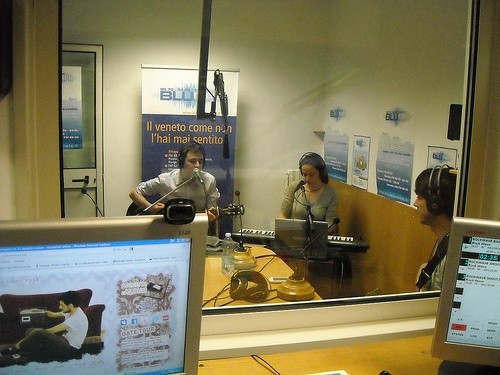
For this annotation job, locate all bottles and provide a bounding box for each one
[221,233,234,275]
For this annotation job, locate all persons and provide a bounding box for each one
[412,167,458,295]
[277,150,339,232]
[0,292,88,366]
[128,144,220,221]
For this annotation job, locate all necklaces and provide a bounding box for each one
[305,182,324,192]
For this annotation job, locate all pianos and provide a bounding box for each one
[231,228,371,297]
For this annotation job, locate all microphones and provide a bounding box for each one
[193,167,204,182]
[289,216,339,279]
[81,188,105,216]
[234,189,246,251]
[297,178,307,188]
[224,126,230,159]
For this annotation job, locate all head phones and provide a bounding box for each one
[427,161,450,214]
[299,150,326,170]
[177,142,200,159]
[229,268,272,300]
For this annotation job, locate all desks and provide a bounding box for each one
[202,244,323,307]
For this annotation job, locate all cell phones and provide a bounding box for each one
[269,276,288,283]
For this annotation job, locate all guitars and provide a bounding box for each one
[125,198,245,218]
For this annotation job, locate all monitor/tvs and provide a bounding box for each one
[429,216,500,374]
[0,210,208,375]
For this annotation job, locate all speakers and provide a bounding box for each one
[447,103,462,140]
